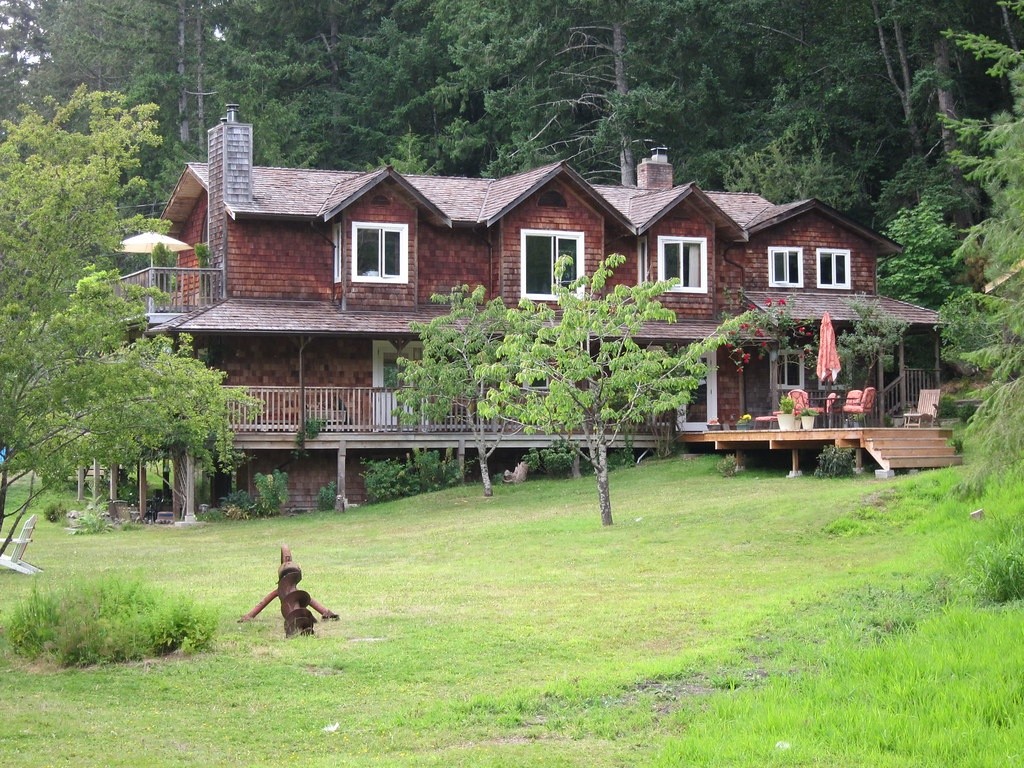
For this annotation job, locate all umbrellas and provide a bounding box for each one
[114,231,193,268]
[817,311,841,395]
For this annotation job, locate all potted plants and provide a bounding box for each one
[776,394,795,430]
[795,407,820,430]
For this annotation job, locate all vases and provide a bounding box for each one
[735,423,751,430]
[707,424,722,431]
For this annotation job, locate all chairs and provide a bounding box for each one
[789,389,825,428]
[753,411,783,429]
[808,393,836,428]
[834,390,863,428]
[843,387,876,428]
[0,514,45,576]
[902,389,942,429]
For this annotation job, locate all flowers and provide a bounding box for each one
[707,417,722,424]
[737,414,751,423]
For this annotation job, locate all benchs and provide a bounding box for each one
[307,410,347,433]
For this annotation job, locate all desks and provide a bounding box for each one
[807,397,859,428]
